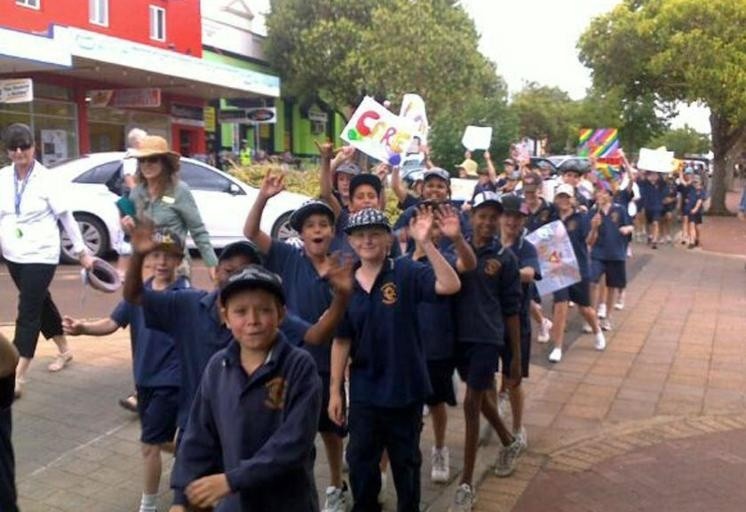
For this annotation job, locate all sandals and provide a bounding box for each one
[120,392,138,411]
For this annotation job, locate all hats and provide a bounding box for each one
[219,263,286,305]
[87,260,122,292]
[343,208,390,234]
[334,158,612,207]
[220,241,264,265]
[289,199,335,229]
[143,231,182,255]
[123,136,180,171]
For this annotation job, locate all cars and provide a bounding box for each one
[43,147,324,263]
[683,153,714,200]
[448,156,638,201]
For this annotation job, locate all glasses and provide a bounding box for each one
[7,143,32,151]
[138,157,160,163]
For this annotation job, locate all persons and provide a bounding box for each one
[121,127,149,191]
[0,122,95,400]
[122,200,354,512]
[114,136,222,416]
[61,231,194,511]
[169,264,323,511]
[244,133,642,510]
[634,158,708,249]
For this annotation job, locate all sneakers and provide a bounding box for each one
[537,318,552,343]
[430,446,449,482]
[494,435,527,476]
[48,352,72,371]
[15,379,26,397]
[323,481,353,512]
[583,299,623,349]
[447,483,477,512]
[549,348,562,361]
